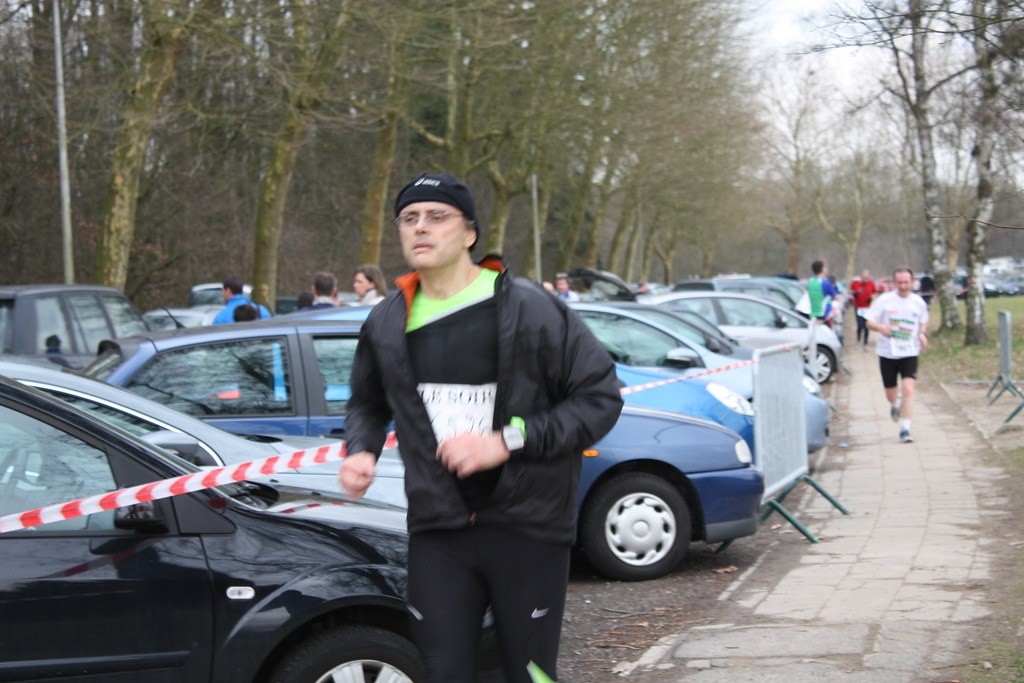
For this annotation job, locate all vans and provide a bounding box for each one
[0,284,155,376]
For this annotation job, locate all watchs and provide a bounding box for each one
[502,425,524,451]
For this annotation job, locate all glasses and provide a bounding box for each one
[394,210,464,225]
[555,272,568,277]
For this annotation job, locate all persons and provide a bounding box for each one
[634,280,650,295]
[866,267,929,443]
[212,263,389,326]
[807,260,935,352]
[550,272,580,302]
[339,173,624,683]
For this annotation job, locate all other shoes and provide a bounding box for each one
[898,427,914,443]
[890,403,899,423]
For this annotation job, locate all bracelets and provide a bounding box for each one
[920,329,926,334]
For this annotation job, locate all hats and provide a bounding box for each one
[393,171,478,221]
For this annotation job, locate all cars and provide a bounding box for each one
[0,377,441,683]
[0,357,413,512]
[135,267,1022,458]
[74,316,766,583]
